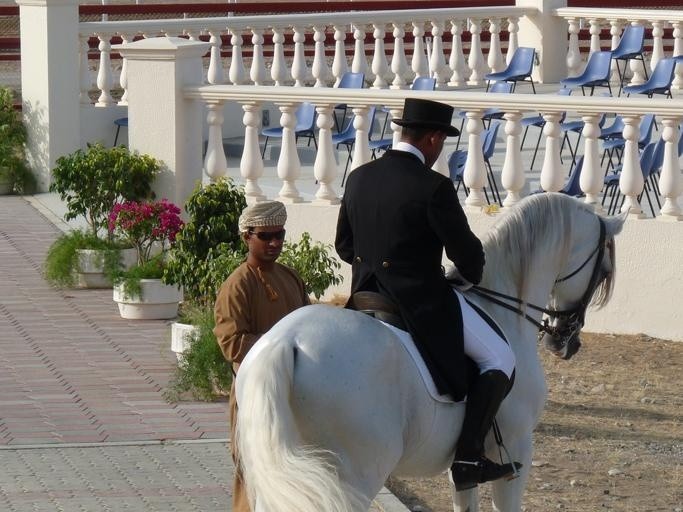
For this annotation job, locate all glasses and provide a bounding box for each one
[249,229,285,241]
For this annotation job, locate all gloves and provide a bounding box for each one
[445,267,474,292]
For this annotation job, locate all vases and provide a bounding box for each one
[110,273,181,320]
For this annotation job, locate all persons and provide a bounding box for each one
[212,201,312,512]
[334,125,523,492]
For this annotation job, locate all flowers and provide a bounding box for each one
[105,200,187,301]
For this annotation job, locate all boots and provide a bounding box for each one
[450,455,523,492]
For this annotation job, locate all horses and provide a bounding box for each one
[228,187,633,512]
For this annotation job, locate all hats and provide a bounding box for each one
[391,99,460,137]
[237,200,286,232]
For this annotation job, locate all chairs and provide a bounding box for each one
[113,117,128,145]
[261,24,683,219]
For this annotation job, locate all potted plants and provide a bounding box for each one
[43,142,157,291]
[1,84,37,197]
[163,175,344,400]
